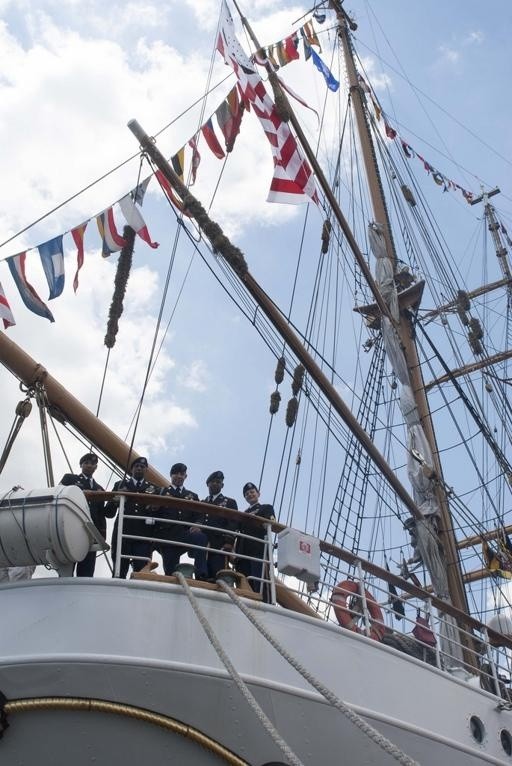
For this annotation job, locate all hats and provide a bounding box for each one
[170,463,187,475]
[80,454,98,464]
[131,457,147,469]
[243,482,257,496]
[205,471,224,484]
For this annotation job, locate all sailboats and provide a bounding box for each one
[1,1,512,766]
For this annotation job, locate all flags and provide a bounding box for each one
[309,46,340,93]
[215,0,320,207]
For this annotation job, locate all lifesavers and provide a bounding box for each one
[332,581,384,644]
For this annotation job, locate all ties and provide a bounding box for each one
[176,487,181,494]
[136,482,141,488]
[208,496,213,504]
[87,478,91,489]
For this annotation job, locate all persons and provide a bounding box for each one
[151,463,217,583]
[200,471,238,584]
[58,453,106,577]
[104,457,159,579]
[235,483,275,604]
